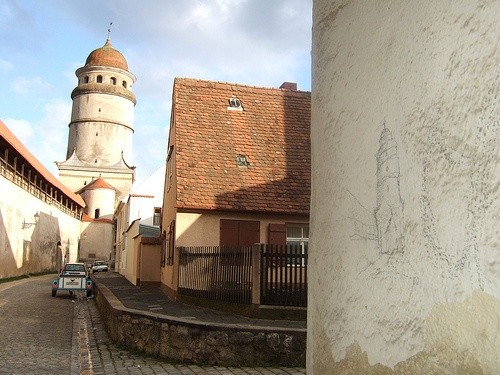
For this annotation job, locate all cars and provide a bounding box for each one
[92,260,108,273]
[51,262,93,297]
